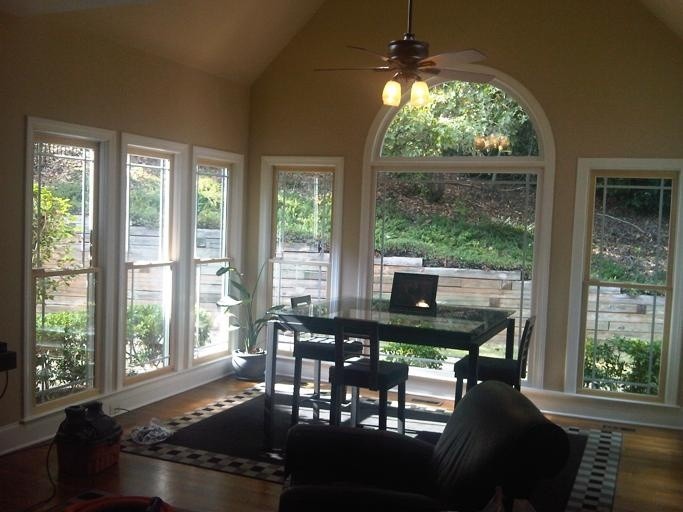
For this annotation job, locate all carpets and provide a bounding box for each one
[120,381,624,512]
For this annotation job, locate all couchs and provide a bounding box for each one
[276,378,569,512]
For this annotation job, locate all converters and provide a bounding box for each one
[0,351,16,371]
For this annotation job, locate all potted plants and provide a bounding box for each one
[214,256,288,382]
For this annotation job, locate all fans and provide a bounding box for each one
[311,0,495,85]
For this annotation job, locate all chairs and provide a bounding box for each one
[451,316,536,410]
[290,294,362,427]
[326,316,408,435]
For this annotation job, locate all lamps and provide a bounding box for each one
[380,69,431,107]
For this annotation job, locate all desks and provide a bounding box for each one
[263,296,516,457]
